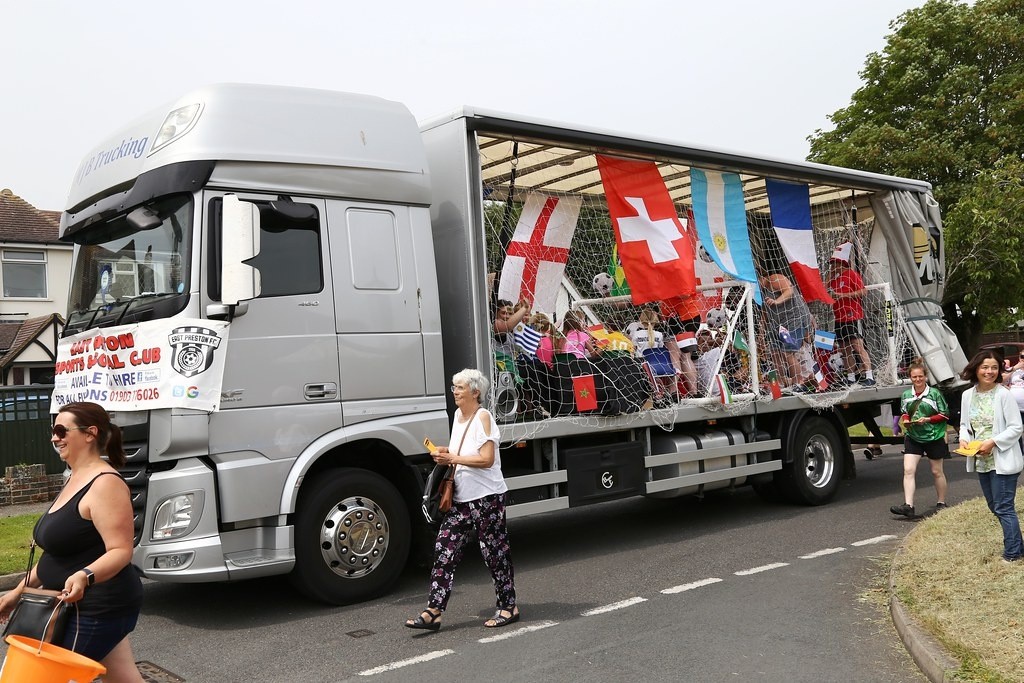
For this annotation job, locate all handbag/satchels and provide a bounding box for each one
[438,477,452,512]
[421,464,449,523]
[2,593,67,645]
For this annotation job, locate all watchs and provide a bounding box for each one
[81,569,94,586]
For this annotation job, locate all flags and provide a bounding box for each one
[594,154,763,305]
[571,375,597,412]
[769,370,782,399]
[733,331,751,357]
[765,177,836,304]
[497,194,582,315]
[716,374,733,405]
[815,330,836,351]
[514,322,541,354]
[587,323,609,347]
[778,327,795,345]
[812,364,828,389]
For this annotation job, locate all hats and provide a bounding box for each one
[826,240,853,266]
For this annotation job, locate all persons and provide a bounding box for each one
[0,402,147,683]
[494,242,873,411]
[864,430,883,460]
[892,403,904,436]
[890,358,948,517]
[404,368,518,629]
[953,351,1024,561]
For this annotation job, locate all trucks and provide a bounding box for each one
[50,84,941,607]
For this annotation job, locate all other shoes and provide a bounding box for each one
[864,447,874,460]
[792,379,817,394]
[814,380,843,392]
[523,410,548,421]
[652,397,671,409]
[667,391,682,405]
[688,392,704,399]
[890,503,915,517]
[857,379,878,388]
[873,447,882,455]
[932,502,947,516]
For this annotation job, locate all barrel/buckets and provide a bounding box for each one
[0,592,107,683]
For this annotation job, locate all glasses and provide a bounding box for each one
[51,424,104,439]
[450,385,477,394]
[1020,355,1024,359]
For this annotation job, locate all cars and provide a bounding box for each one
[979,342,1024,368]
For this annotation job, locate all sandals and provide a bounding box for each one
[484,603,520,627]
[404,610,441,631]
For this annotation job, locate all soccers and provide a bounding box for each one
[625,322,645,336]
[706,307,729,330]
[592,272,616,294]
[827,353,845,371]
